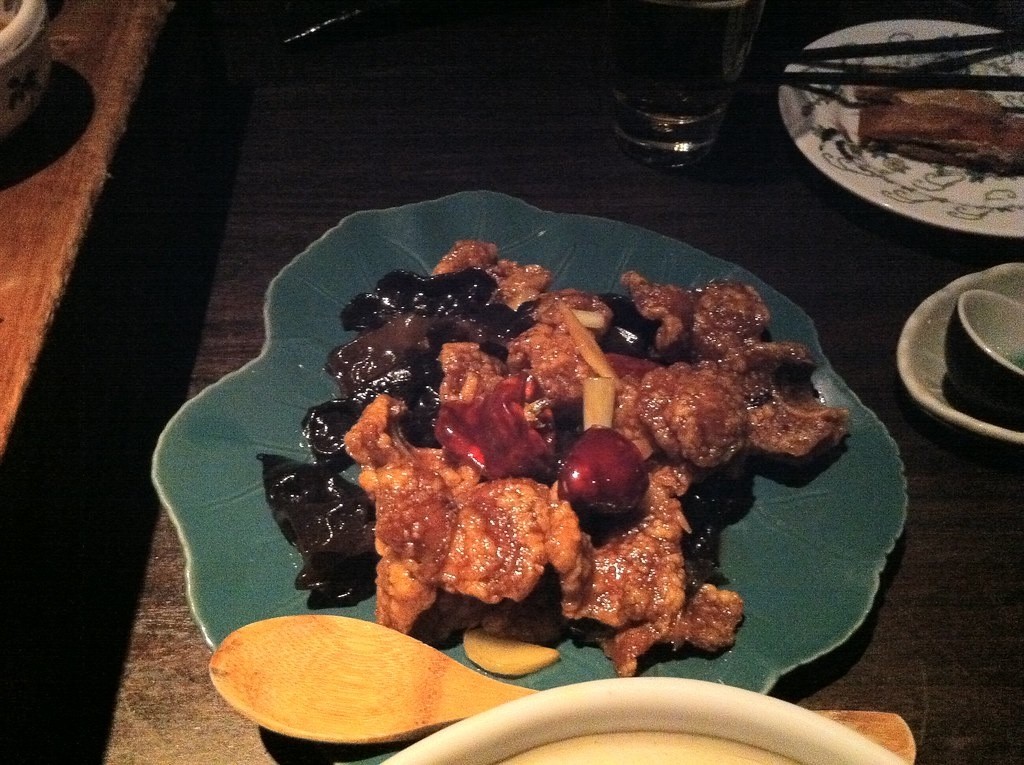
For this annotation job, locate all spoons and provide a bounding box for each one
[206,612,918,764]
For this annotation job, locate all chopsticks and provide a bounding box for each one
[764,30,1024,90]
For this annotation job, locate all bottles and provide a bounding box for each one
[616,0,765,170]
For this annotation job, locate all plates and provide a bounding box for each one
[779,18,1024,238]
[149,188,904,731]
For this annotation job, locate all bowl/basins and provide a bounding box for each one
[383,674,908,765]
[0,0,55,139]
[943,291,1024,407]
[894,261,1024,450]
